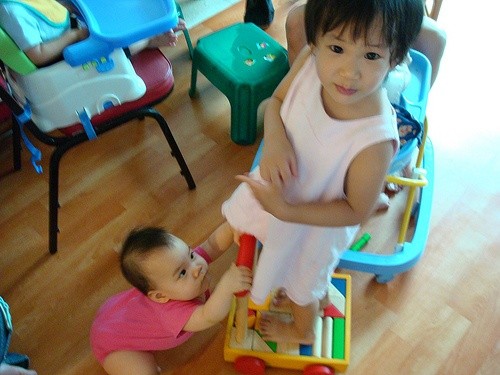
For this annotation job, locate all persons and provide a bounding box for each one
[0,0,187,65]
[91,220,253,375]
[221,1,424,342]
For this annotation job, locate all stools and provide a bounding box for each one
[187,22,289,146]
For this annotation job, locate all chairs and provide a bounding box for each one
[0,0,197,255]
